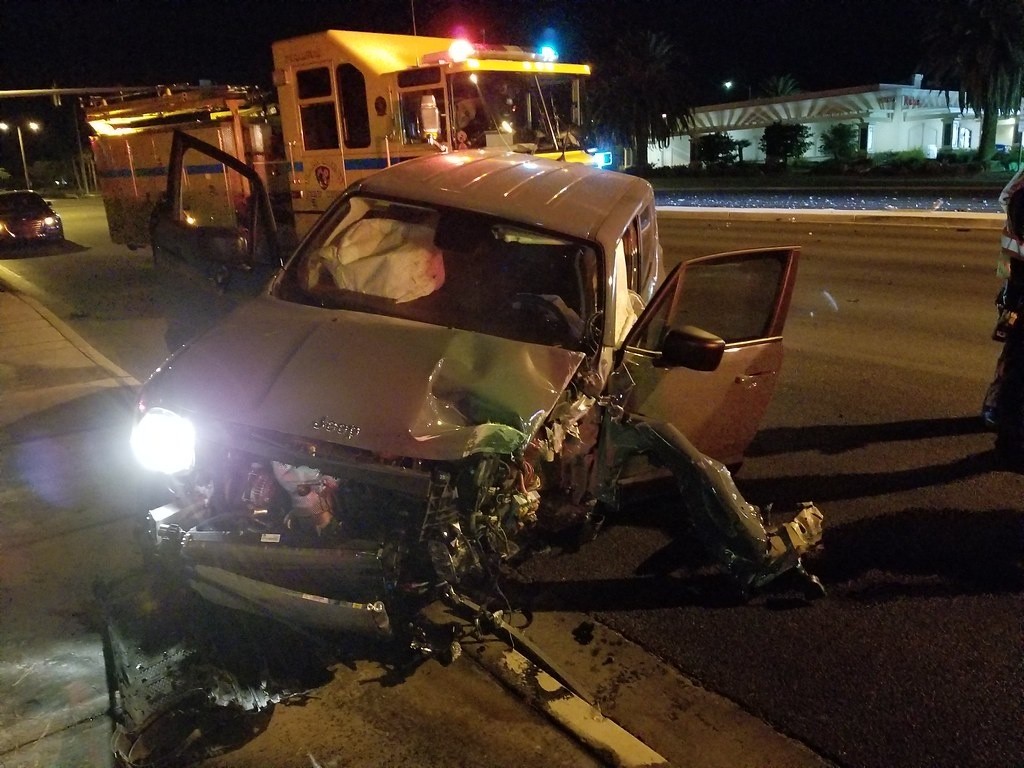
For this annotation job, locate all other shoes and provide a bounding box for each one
[981,406,1000,430]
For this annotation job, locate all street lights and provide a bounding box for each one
[1,116,44,189]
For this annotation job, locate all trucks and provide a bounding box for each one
[80,28,613,312]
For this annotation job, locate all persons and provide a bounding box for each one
[978,169,1024,463]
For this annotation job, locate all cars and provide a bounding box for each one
[0,189,66,251]
[106,130,826,661]
